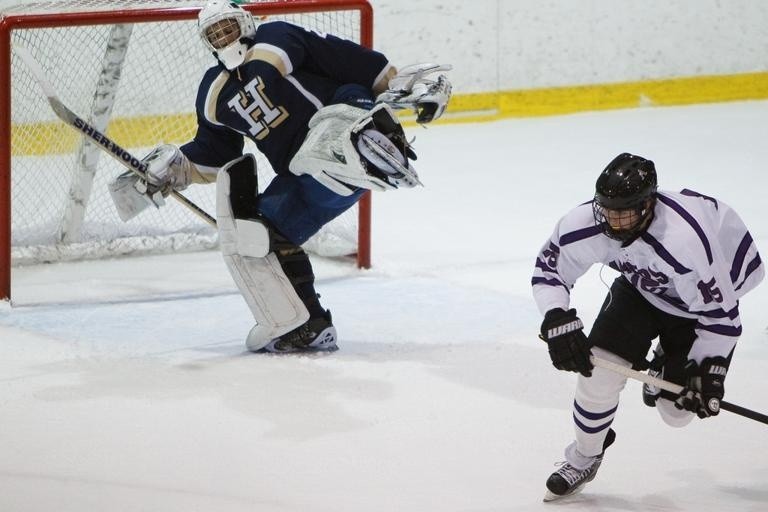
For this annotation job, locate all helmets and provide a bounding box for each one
[198,0,256,71]
[593,152,658,243]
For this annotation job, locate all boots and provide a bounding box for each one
[642,344,667,408]
[547,428,617,496]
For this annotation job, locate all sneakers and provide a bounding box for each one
[265,310,340,352]
[357,130,418,189]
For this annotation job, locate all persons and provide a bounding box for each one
[107,0,453,354]
[530,153,767,497]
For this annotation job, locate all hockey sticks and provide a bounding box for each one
[12,43,218,229]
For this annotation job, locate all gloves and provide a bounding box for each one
[676,358,727,418]
[538,308,594,377]
